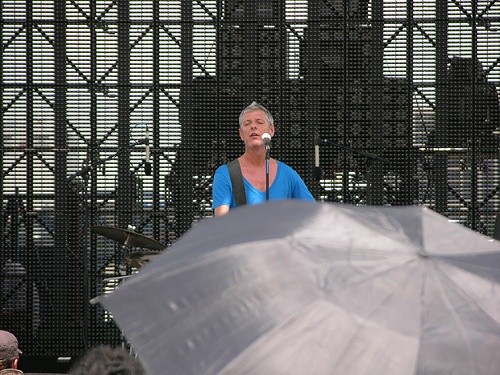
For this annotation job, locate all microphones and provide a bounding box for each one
[261,133,271,145]
[313,125,322,178]
[145,125,151,176]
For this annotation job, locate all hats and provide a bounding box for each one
[0,330,23,365]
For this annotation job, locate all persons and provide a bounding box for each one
[0,329,24,375]
[212,102,315,219]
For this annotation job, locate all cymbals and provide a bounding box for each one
[92,224,165,250]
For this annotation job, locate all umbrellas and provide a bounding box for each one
[97,200,500,375]
